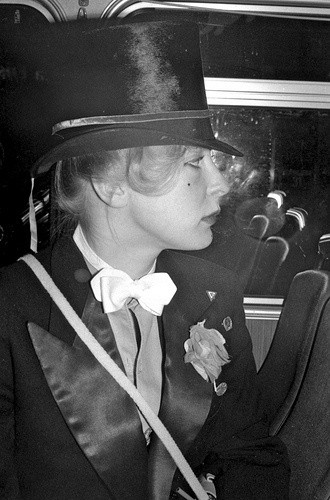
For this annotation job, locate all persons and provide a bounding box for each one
[0,12,291,500]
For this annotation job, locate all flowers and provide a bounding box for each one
[185,320,232,395]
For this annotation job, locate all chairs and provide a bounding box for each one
[257,268,330,500]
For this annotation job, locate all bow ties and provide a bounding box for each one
[72,223,177,316]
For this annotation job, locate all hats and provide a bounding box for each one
[27,18,244,179]
